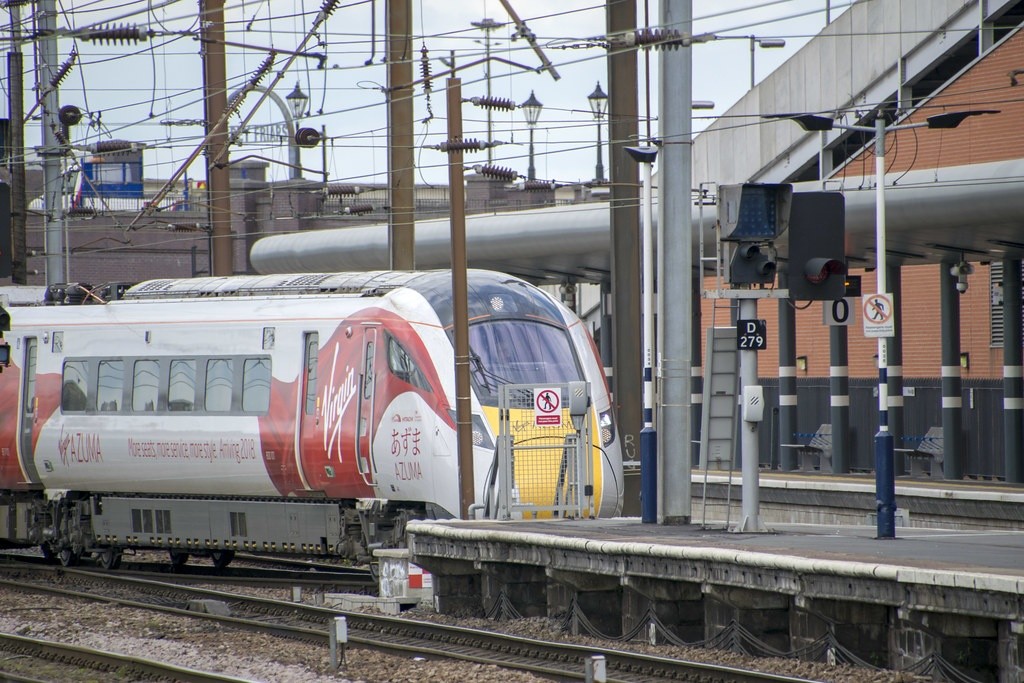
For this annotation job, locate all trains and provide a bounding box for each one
[1,269,626,584]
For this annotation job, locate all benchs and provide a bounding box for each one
[893,425,944,478]
[779,424,832,471]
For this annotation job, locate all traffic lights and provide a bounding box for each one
[716,184,788,287]
[788,194,849,302]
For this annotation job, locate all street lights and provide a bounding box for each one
[748,37,784,89]
[521,92,541,180]
[287,81,309,180]
[760,109,1001,542]
[588,80,610,183]
[625,146,659,527]
